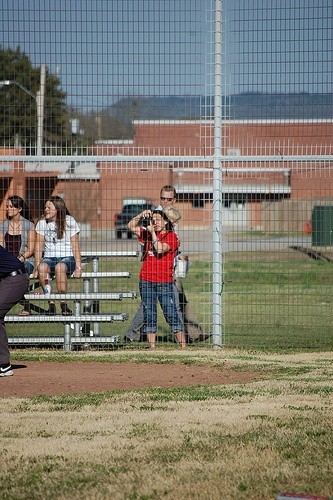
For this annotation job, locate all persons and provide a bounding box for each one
[0,195,36,316]
[127,210,186,349]
[122,186,210,343]
[34,195,83,316]
[0,246,29,377]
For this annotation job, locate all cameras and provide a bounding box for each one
[142,214,153,227]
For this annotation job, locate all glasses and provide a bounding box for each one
[160,197,173,202]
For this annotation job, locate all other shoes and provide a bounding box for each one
[195,333,211,343]
[60,303,73,315]
[45,308,57,316]
[124,333,146,342]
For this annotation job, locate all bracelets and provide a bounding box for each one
[76,267,82,270]
[21,255,25,261]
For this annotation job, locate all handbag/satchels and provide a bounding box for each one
[175,255,192,279]
[138,242,144,262]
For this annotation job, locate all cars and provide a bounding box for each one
[114,203,156,239]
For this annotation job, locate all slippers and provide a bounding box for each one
[18,309,31,316]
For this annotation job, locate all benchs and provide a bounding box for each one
[3,250,140,351]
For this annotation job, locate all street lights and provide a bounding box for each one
[0,80,36,101]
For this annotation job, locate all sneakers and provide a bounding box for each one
[0,365,14,377]
